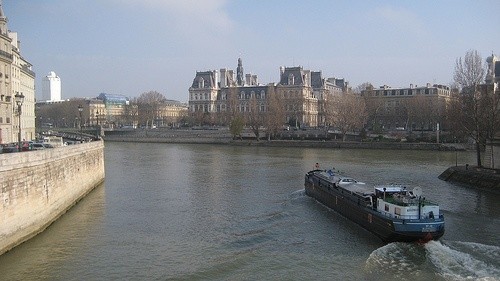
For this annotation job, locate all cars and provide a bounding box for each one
[0,137,50,153]
[42,131,92,142]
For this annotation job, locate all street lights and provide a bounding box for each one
[15,91,25,150]
[78,104,83,143]
[96,111,100,140]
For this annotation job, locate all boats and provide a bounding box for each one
[303,163,445,246]
